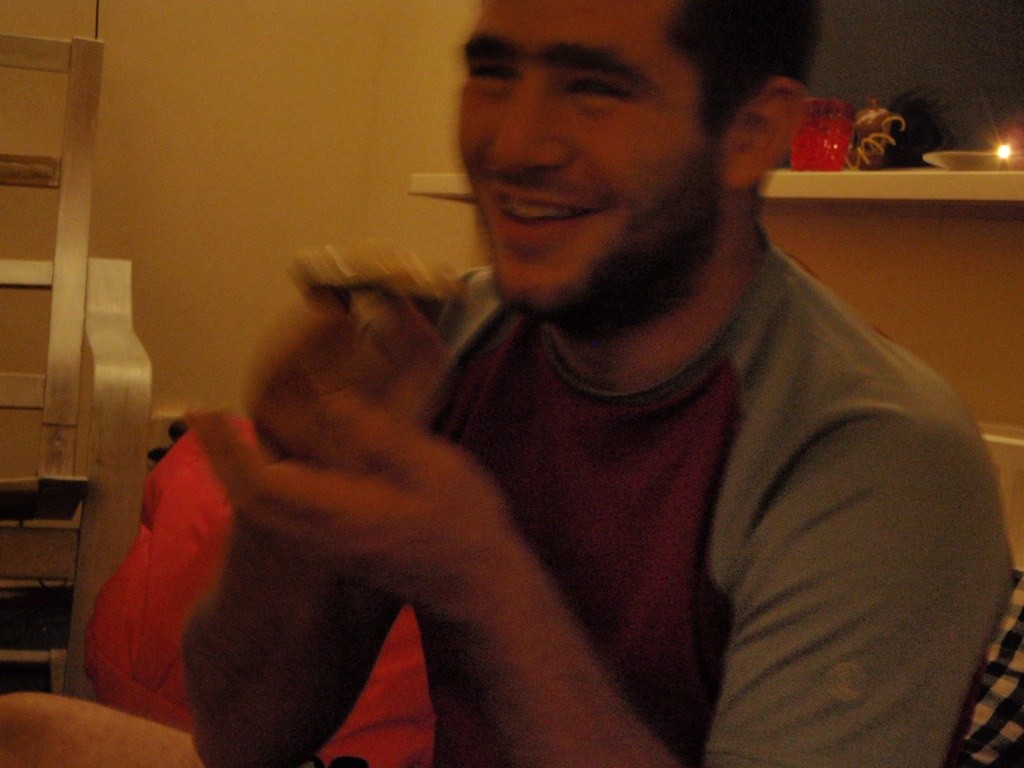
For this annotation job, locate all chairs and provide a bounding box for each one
[0,32,152,703]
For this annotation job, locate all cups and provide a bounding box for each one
[791,99,854,170]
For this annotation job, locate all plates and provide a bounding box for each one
[922,151,1024,170]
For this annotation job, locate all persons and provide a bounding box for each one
[185,0,1014,767]
[1,413,438,768]
